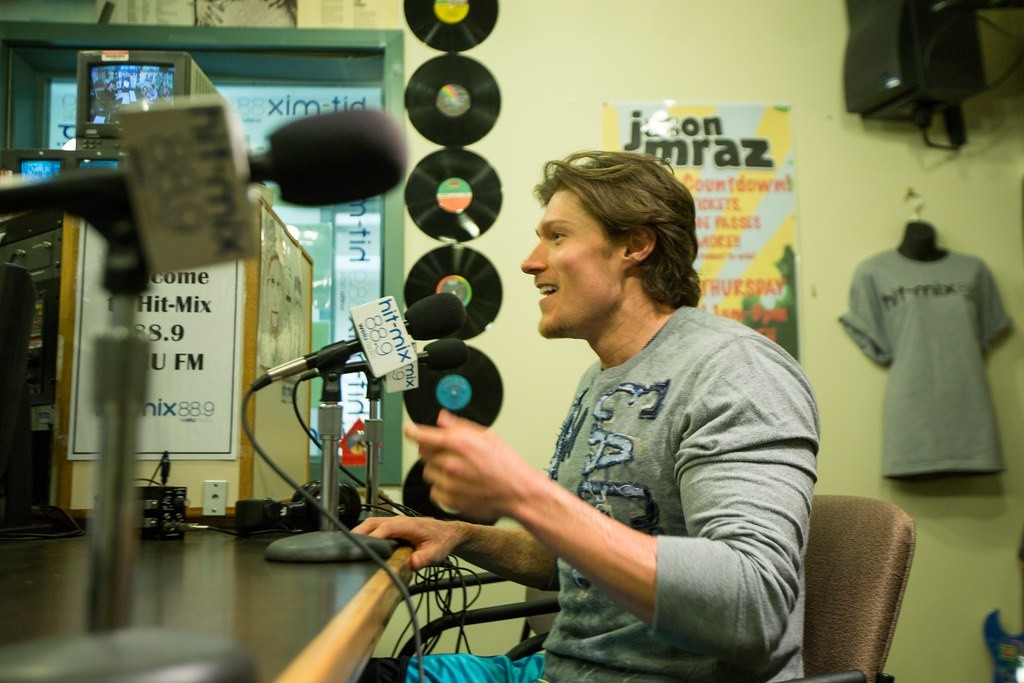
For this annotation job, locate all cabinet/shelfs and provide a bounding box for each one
[50,193,312,517]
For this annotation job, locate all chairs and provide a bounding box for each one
[398,496,915,682]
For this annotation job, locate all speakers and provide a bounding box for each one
[845,0,987,128]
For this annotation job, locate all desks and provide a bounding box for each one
[0,510,414,683]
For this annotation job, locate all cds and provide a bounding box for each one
[402,1,502,525]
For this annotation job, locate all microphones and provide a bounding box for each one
[0,109,403,223]
[253,294,464,395]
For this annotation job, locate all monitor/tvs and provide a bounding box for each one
[78,50,219,137]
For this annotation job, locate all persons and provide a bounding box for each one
[102,80,117,103]
[346,153,821,683]
[841,222,1011,478]
[145,84,158,101]
